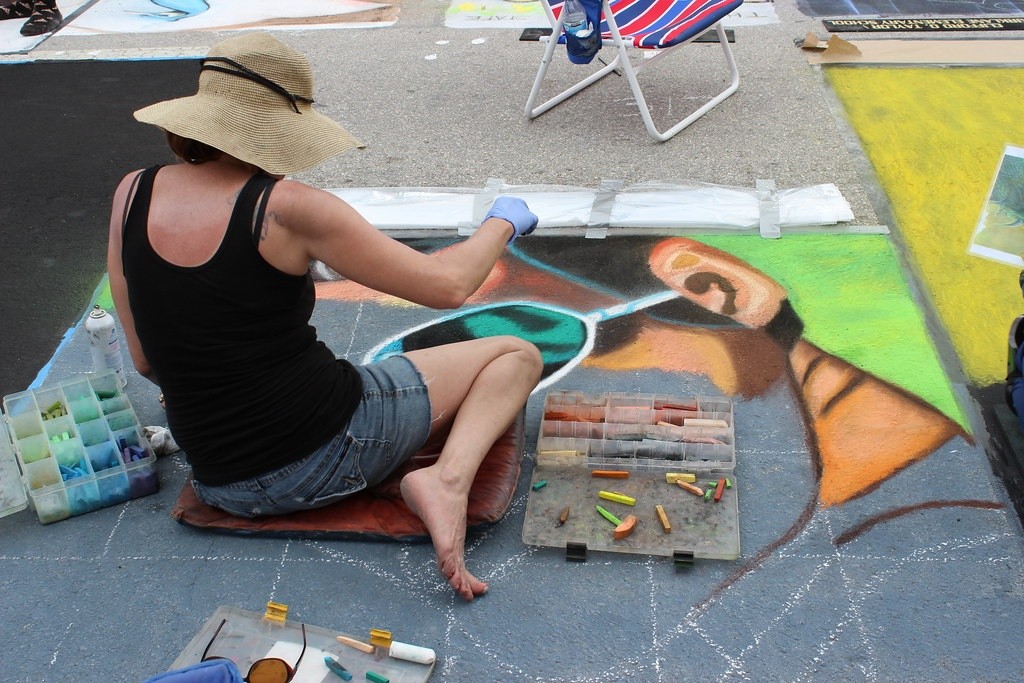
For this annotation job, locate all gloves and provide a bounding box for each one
[481,197,536,246]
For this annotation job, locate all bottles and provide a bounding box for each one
[86,304,127,389]
[562,0,587,52]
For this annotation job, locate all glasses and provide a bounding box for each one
[199,617,308,683]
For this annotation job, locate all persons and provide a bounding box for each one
[105,30,546,604]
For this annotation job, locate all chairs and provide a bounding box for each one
[524,0,744,142]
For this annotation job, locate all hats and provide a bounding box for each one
[133,30,365,178]
